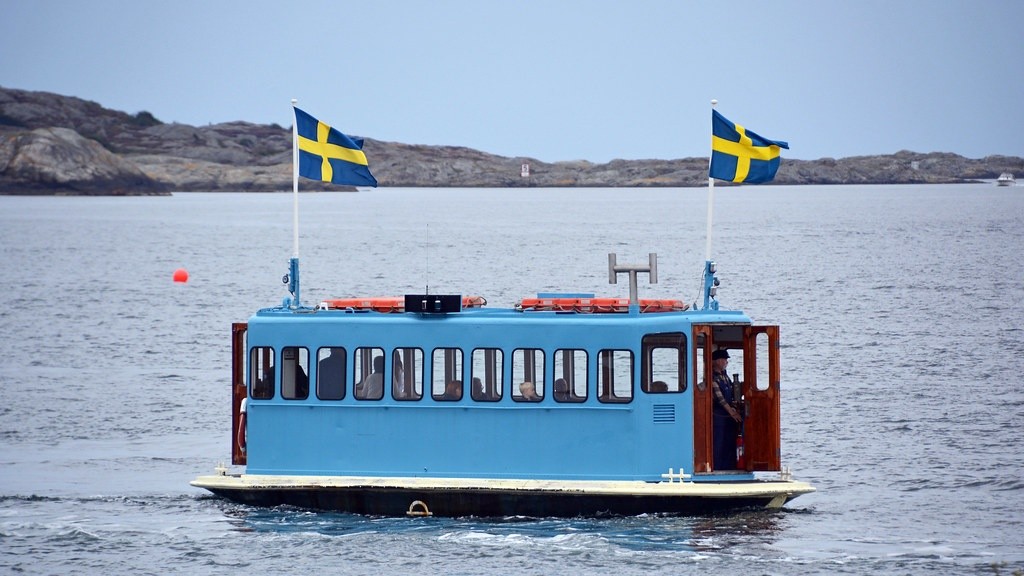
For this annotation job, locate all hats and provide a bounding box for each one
[712,350,733,361]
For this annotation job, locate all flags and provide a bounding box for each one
[708,108,789,185]
[293,106,379,188]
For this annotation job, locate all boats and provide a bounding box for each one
[996,172,1016,186]
[190,253,816,517]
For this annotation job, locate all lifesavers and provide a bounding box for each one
[237,398,247,454]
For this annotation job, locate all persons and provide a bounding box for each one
[265,349,308,398]
[650,381,668,392]
[442,380,461,400]
[712,349,743,470]
[519,382,539,402]
[319,347,345,399]
[555,379,571,400]
[362,356,383,399]
[473,378,490,400]
[394,349,403,399]
